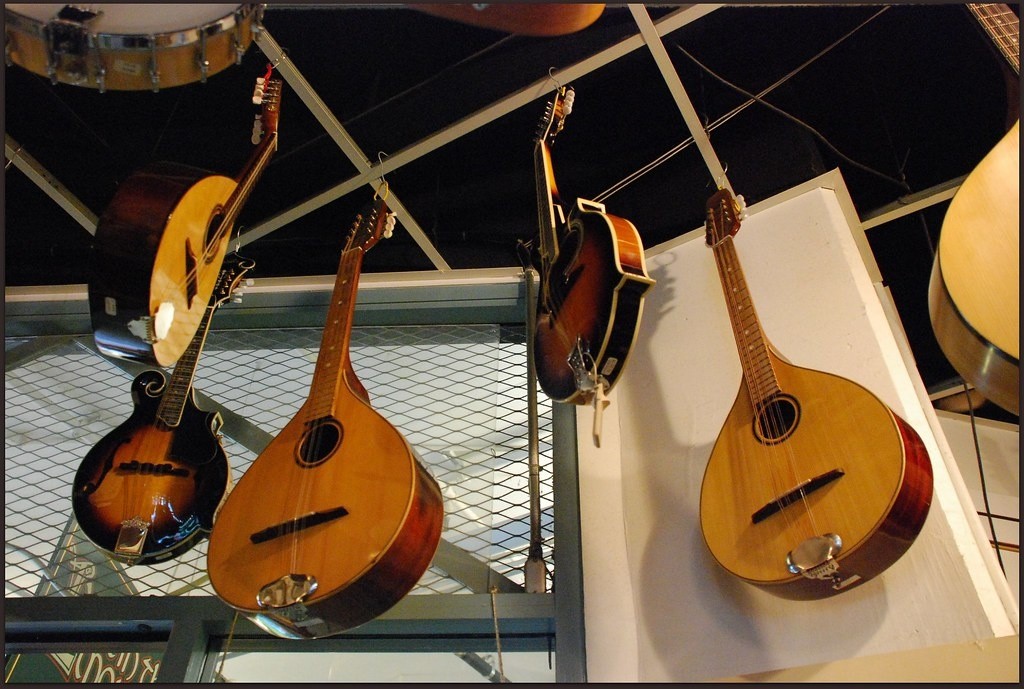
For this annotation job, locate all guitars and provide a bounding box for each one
[519,62,658,403]
[406,0,606,36]
[700,189,932,601]
[207,149,443,639]
[926,0,1024,418]
[90,57,284,366]
[71,250,255,565]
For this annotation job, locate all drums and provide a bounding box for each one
[3,1,265,91]
